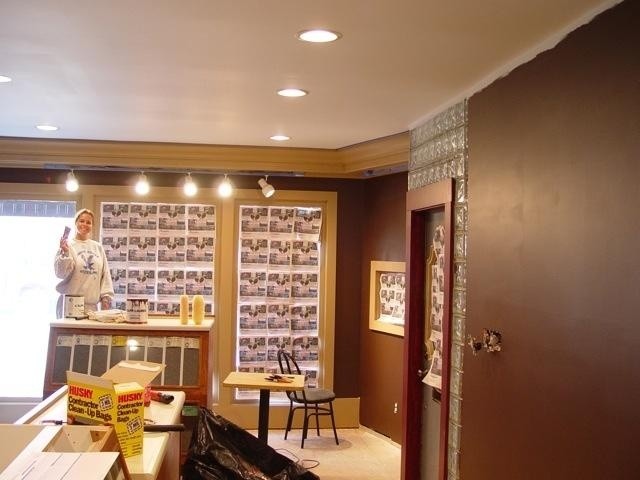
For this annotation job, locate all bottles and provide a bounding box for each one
[179,294,189,325]
[192,294,205,324]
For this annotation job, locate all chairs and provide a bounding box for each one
[278,349,340,445]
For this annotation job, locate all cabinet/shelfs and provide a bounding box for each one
[33,315,213,415]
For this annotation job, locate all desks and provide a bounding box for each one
[221,370,306,458]
[9,377,186,479]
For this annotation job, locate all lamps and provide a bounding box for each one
[58,167,283,200]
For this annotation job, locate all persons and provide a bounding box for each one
[51,208,113,320]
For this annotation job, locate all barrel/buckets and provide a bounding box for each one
[64,294,85,319]
[127,299,148,324]
